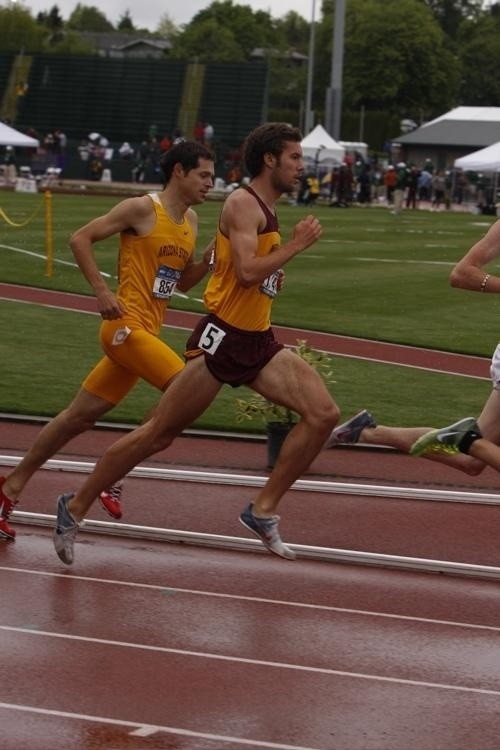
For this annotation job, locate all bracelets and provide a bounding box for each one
[479,273,491,295]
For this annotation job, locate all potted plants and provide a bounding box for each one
[233,338,337,471]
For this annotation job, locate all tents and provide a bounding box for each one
[300,124,344,163]
[449,142,500,204]
[0,122,40,147]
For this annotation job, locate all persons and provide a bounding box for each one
[51,119,345,563]
[0,140,221,543]
[329,151,497,213]
[407,414,499,476]
[0,127,106,180]
[134,121,244,189]
[325,202,499,481]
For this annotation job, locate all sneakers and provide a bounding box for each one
[238,502,296,560]
[409,417,483,457]
[100,480,125,519]
[53,492,79,565]
[324,409,376,448]
[1,476,19,539]
